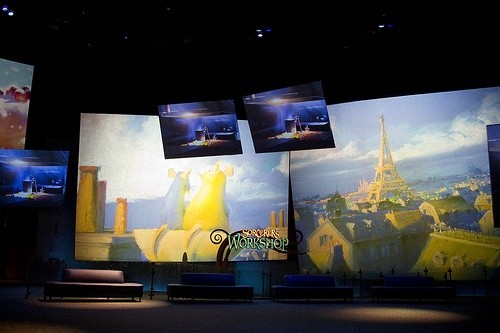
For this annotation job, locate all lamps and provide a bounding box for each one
[256,24,270,38]
[1,0,15,17]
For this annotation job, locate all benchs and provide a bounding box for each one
[269,275,355,305]
[371,275,458,304]
[43,269,144,302]
[166,273,255,302]
